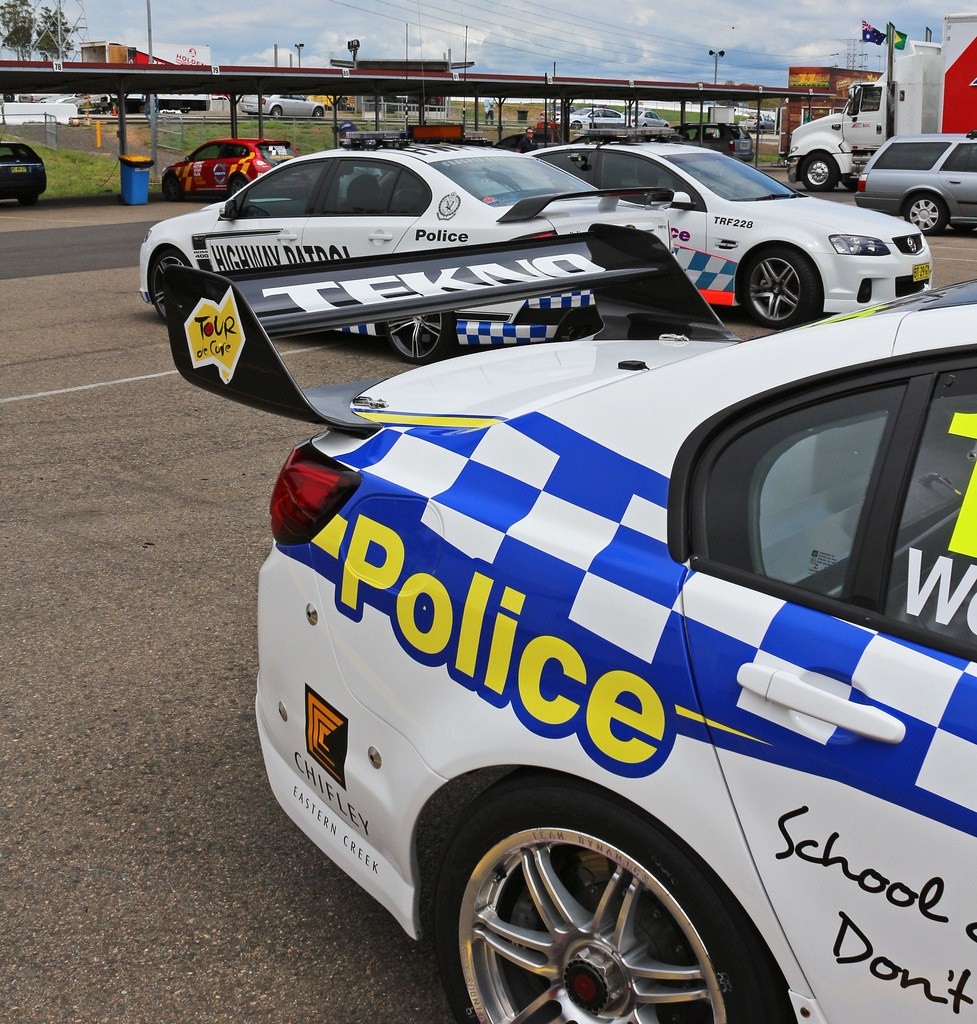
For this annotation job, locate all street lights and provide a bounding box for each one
[709,49,725,122]
[295,43,304,68]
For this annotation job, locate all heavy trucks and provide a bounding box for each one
[783,12,977,194]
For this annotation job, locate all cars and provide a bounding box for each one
[137,135,676,365]
[554,107,627,130]
[523,133,936,331]
[539,104,576,124]
[39,93,111,117]
[0,141,48,206]
[158,223,977,1024]
[239,95,326,120]
[621,109,670,128]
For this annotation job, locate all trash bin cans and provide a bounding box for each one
[117,153,155,205]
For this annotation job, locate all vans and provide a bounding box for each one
[853,130,977,237]
[654,123,755,164]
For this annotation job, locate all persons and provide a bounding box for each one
[518,125,540,157]
[484,97,497,126]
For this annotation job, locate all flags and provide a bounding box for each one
[884,28,908,51]
[861,20,888,47]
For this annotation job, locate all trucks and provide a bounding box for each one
[737,114,778,134]
